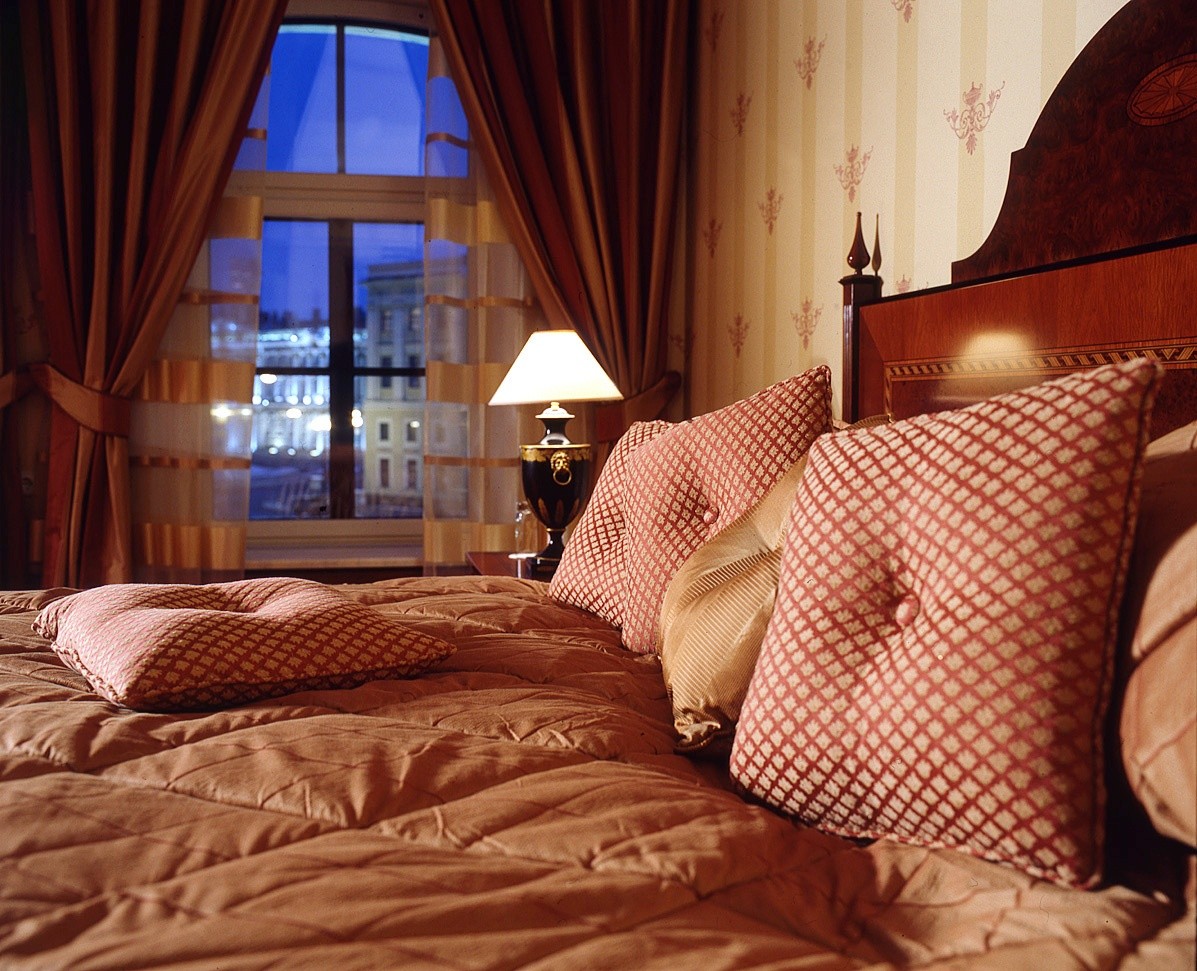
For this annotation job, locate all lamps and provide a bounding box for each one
[487,328,623,564]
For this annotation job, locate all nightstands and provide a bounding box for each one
[467,550,568,579]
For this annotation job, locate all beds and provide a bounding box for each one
[0,0,1197,971]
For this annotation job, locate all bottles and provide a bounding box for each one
[514,501,540,554]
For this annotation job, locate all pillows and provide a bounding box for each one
[546,372,1197,911]
[29,578,456,705]
